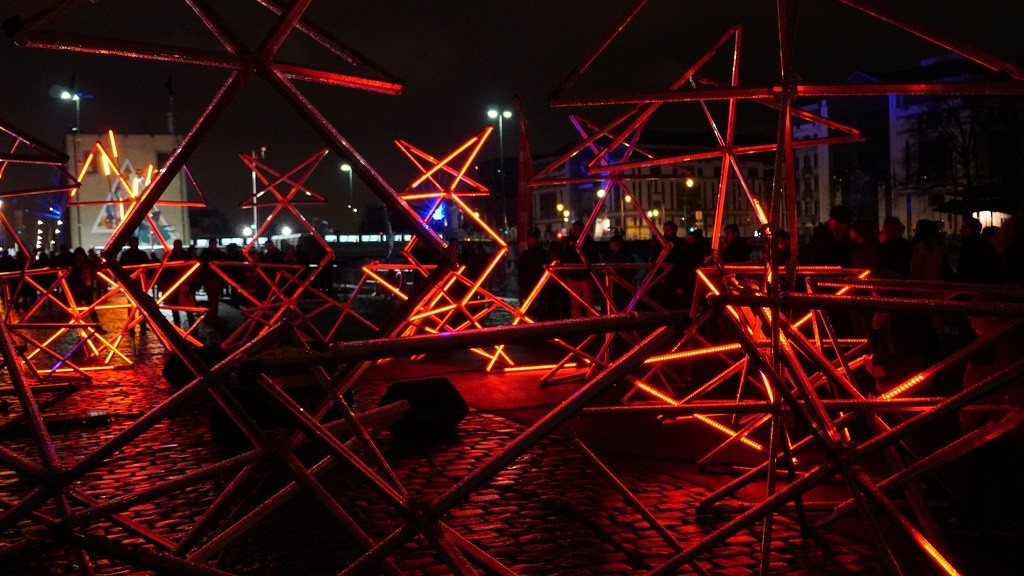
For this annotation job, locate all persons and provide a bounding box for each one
[0,237,495,335]
[517,206,1024,391]
